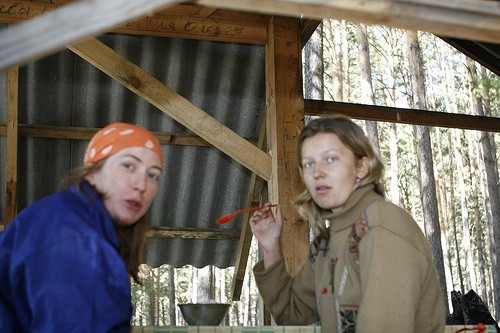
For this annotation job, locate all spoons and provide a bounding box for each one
[215,204,279,224]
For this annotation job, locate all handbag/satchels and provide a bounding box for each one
[448,289,500,333]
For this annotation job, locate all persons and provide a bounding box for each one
[249,116,446,333]
[0,122,164,333]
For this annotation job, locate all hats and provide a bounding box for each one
[83,123,164,169]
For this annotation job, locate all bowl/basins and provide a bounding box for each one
[178,303,231,326]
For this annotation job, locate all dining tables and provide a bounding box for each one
[131,326,322,333]
